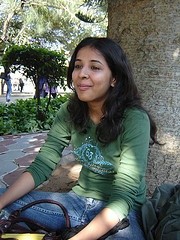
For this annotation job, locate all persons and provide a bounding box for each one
[1,73,60,103]
[0,36,166,240]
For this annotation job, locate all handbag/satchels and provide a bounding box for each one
[0,198,73,240]
